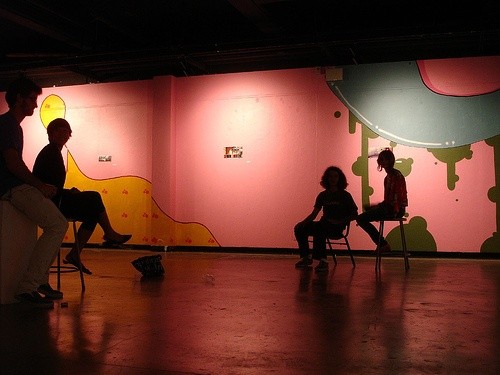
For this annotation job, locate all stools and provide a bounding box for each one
[49,220,86,292]
[375,218,409,273]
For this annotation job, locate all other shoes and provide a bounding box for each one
[39,282,63,299]
[102,234,132,246]
[315,260,328,271]
[31,293,53,308]
[373,245,390,253]
[295,257,313,266]
[63,255,93,275]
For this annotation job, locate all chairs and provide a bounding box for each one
[308,219,357,269]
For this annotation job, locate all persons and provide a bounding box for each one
[356,150,408,254]
[293,167,359,271]
[0,79,69,311]
[32,118,132,274]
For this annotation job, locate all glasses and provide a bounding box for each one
[24,96,37,101]
